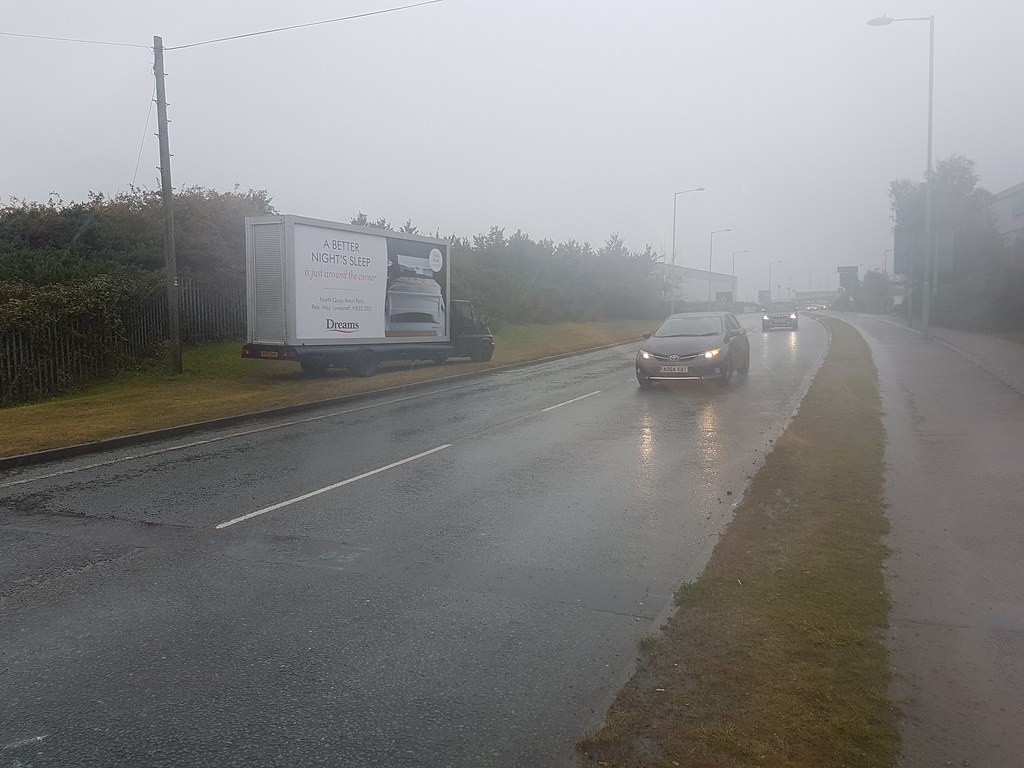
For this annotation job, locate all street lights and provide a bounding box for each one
[885,249,890,272]
[733,250,750,277]
[671,188,705,317]
[769,261,784,297]
[868,15,934,339]
[709,228,731,311]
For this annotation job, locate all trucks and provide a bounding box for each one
[240,211,498,371]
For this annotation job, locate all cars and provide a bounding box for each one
[635,310,750,389]
[761,298,800,331]
[807,300,830,310]
